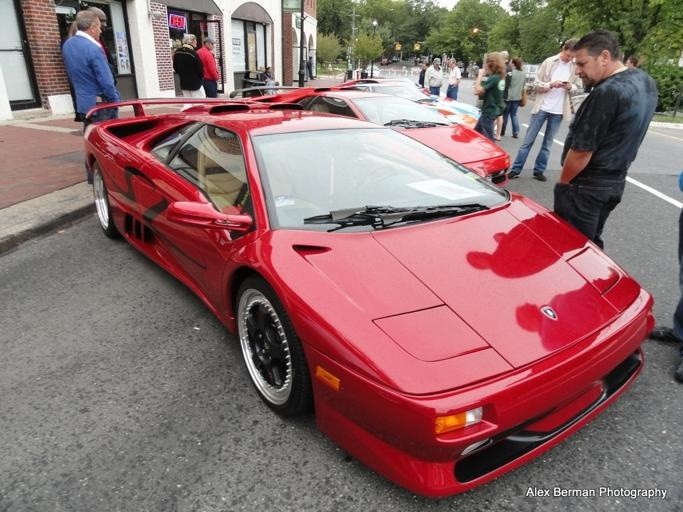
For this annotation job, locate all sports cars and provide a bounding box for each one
[83,98,654,501]
[264,88,510,188]
[340,83,484,130]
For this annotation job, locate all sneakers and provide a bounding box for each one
[508,171,521,178]
[534,172,546,181]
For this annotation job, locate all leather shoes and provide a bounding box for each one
[650,327,681,343]
[675,360,683,382]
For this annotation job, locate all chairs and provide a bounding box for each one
[198,136,250,229]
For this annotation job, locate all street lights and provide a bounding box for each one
[369,19,378,78]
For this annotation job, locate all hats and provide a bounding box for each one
[204,38,215,43]
[433,58,441,64]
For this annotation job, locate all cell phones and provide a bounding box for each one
[562,81,567,85]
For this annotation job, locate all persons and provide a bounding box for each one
[261,66,275,95]
[67,19,79,38]
[86,7,119,119]
[173,33,206,99]
[196,38,219,97]
[420,29,683,384]
[307,56,314,80]
[61,10,122,184]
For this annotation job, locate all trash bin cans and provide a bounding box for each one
[347,70,352,79]
[242,70,265,97]
[361,73,368,79]
